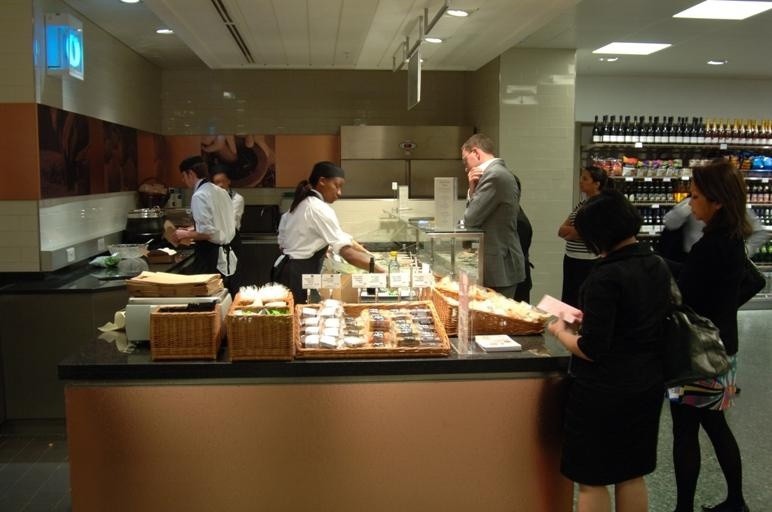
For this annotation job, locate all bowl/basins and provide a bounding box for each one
[116,259,149,274]
[106,243,149,261]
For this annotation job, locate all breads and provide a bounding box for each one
[298,299,441,348]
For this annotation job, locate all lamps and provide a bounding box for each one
[45,11,87,81]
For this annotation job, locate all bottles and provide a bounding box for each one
[622,180,690,234]
[745,183,771,261]
[388,251,400,294]
[367,256,376,295]
[591,114,772,145]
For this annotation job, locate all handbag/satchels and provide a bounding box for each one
[738,257,765,309]
[648,303,728,389]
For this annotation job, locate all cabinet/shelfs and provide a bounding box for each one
[344,157,467,199]
[578,120,772,267]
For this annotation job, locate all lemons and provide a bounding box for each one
[390,250,398,256]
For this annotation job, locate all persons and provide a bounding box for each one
[170,156,390,303]
[558,166,615,310]
[460,133,534,305]
[664,160,766,512]
[665,196,766,258]
[548,190,670,512]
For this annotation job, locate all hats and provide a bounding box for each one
[179,156,203,172]
[312,162,344,177]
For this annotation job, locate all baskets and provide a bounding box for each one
[136,177,170,209]
[151,306,223,360]
[430,286,545,335]
[228,288,300,362]
